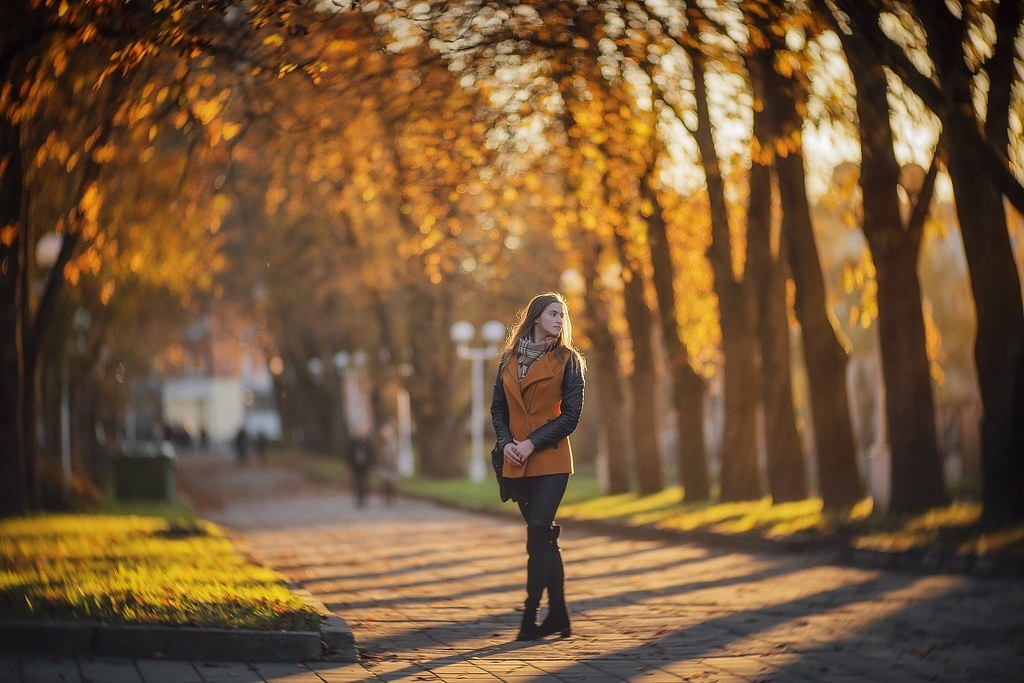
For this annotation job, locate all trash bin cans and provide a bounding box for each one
[112,442,177,502]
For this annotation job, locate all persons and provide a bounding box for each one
[347,425,400,510]
[490,293,586,641]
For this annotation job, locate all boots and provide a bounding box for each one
[538,542,571,639]
[517,520,561,641]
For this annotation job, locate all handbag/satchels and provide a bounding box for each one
[491,441,517,503]
